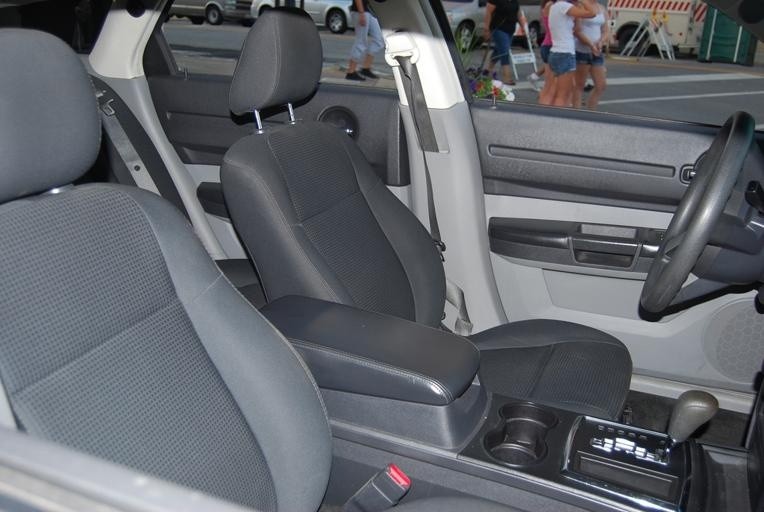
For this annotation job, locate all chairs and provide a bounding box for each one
[1,7,634,512]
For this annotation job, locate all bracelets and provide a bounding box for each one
[358,11,364,14]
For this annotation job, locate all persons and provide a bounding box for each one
[345,0,385,81]
[484,0,526,85]
[530,0,610,110]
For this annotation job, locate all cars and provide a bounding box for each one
[440,0,546,54]
[164,0,252,27]
[250,1,355,35]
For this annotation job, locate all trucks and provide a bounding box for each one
[602,0,708,58]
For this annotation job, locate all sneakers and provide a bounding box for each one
[526,74,541,91]
[345,68,378,80]
